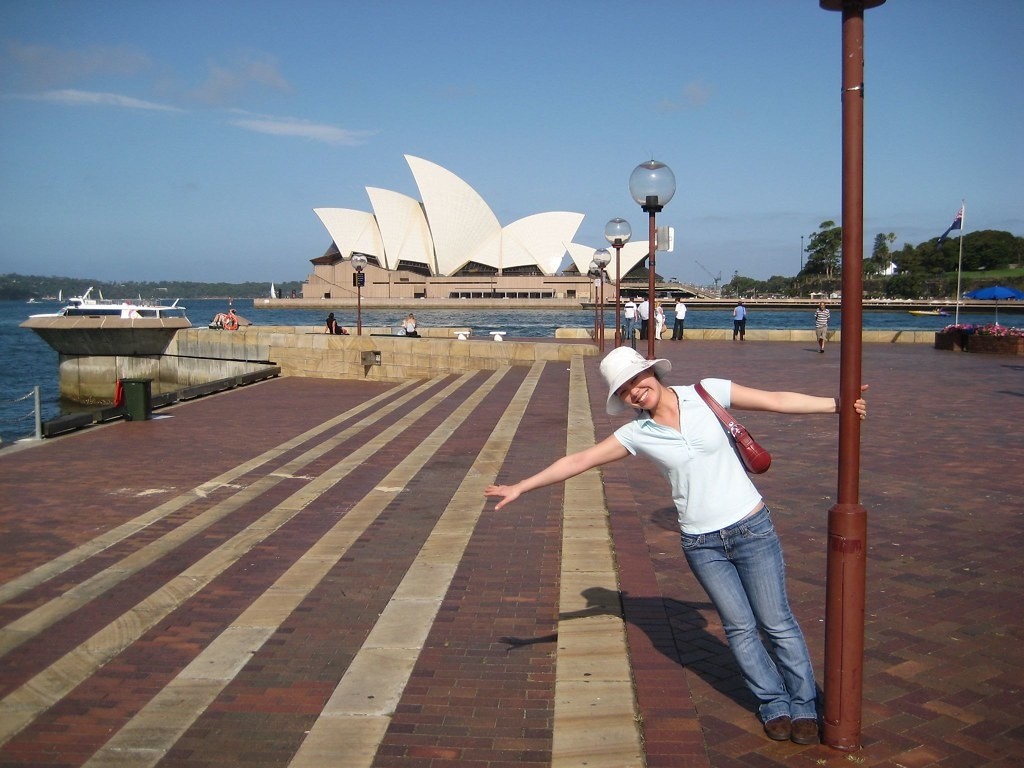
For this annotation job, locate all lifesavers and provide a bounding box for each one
[222,315,238,330]
[129,309,138,320]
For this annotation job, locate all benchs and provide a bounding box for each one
[305,332,350,335]
[370,334,421,338]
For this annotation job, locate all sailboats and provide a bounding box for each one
[58,289,65,303]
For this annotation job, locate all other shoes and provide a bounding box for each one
[820,348,824,353]
[678,337,683,340]
[670,337,676,340]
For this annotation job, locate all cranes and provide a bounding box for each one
[695,260,722,290]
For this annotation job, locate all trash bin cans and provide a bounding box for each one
[118,378,154,421]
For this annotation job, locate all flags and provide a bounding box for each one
[936,206,963,243]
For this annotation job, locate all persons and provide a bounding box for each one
[669,297,687,340]
[483,344,870,745]
[624,295,666,341]
[324,312,347,335]
[815,301,831,352]
[733,301,747,340]
[406,313,417,337]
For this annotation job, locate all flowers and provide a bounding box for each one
[941,324,1024,337]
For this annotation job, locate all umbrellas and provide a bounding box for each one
[962,284,1024,326]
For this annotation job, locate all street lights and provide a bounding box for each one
[351,252,367,334]
[589,247,611,352]
[628,158,677,359]
[603,216,632,347]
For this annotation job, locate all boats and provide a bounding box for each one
[908,309,951,316]
[26,299,42,304]
[28,286,211,328]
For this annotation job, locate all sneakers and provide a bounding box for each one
[791,717,819,745]
[764,716,792,740]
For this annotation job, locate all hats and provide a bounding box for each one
[599,346,672,416]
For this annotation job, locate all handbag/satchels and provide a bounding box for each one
[693,382,772,474]
[743,307,747,321]
[661,323,667,332]
